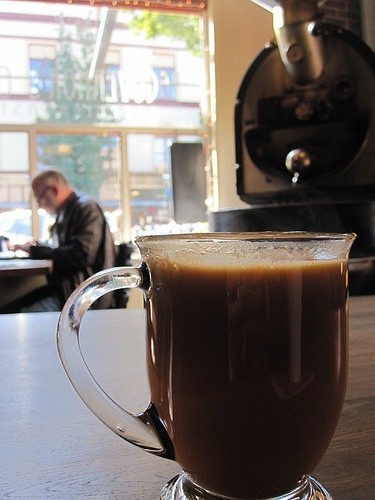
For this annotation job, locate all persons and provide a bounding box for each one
[0,169,118,314]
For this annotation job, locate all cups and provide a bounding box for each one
[56,231,356,500]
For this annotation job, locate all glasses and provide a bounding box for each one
[36,185,50,202]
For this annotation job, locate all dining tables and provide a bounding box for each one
[0,294,375,500]
[0,259,53,312]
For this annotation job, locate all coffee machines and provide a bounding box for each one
[209,0,375,296]
